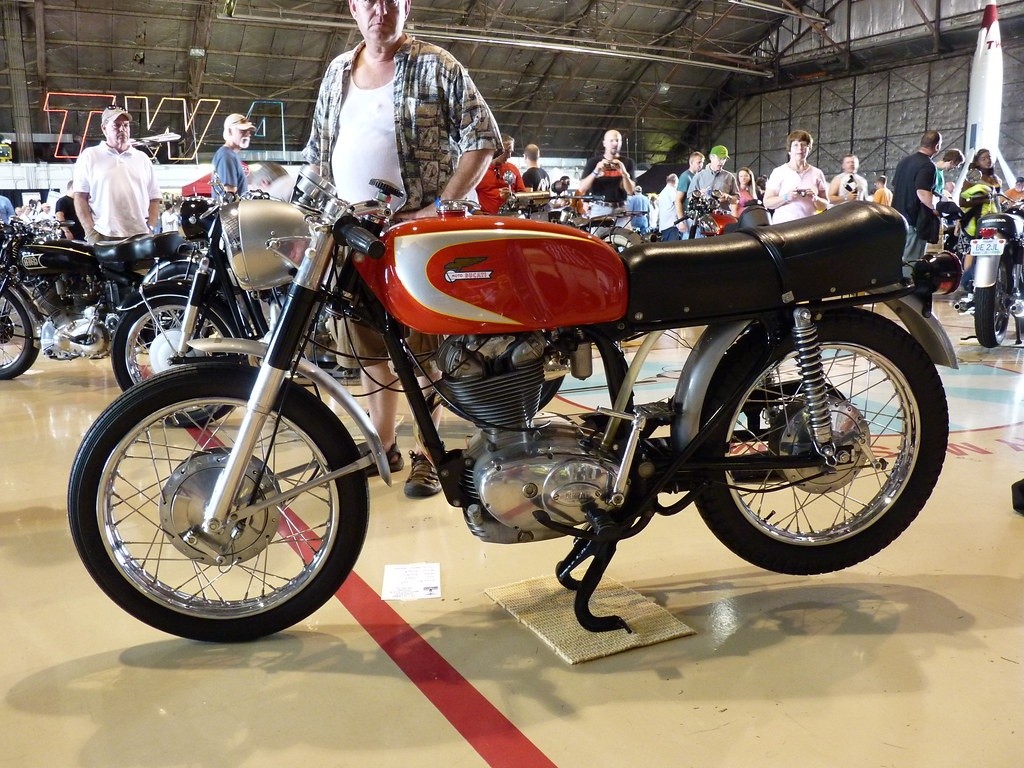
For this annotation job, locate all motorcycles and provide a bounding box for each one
[65,167,959,646]
[960,165,1024,351]
[0,187,664,425]
[672,189,772,239]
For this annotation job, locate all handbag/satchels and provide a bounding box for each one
[917,203,939,245]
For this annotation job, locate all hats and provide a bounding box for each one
[711,145,730,160]
[225,114,257,132]
[634,185,643,192]
[101,104,133,124]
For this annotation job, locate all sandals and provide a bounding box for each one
[356,434,405,475]
[404,449,442,497]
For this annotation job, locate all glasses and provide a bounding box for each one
[105,104,126,111]
[494,167,501,179]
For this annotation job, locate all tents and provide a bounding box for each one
[182,174,213,200]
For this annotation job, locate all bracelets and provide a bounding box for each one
[592,170,598,177]
[470,208,476,214]
[844,194,847,201]
[812,196,818,202]
[435,196,442,209]
[146,223,155,234]
[783,193,790,204]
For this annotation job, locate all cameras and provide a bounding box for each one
[798,189,808,197]
[605,163,620,170]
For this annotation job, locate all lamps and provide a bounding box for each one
[189,46,205,61]
[656,82,671,95]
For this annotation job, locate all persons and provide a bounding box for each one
[162,203,178,233]
[0,181,88,242]
[628,144,768,242]
[872,130,1024,291]
[211,113,257,204]
[251,162,295,205]
[301,0,505,496]
[73,107,164,358]
[579,128,637,252]
[521,143,551,222]
[550,176,572,209]
[828,154,869,208]
[462,133,526,216]
[762,130,830,226]
